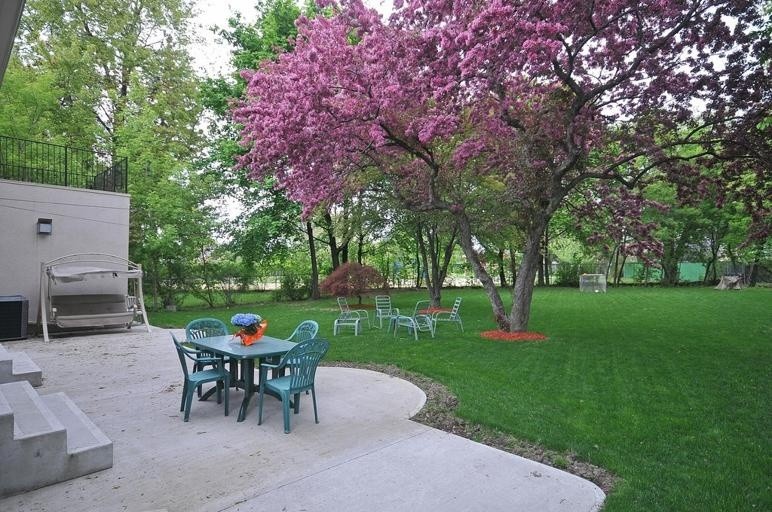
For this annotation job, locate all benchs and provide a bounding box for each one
[37,252,154,344]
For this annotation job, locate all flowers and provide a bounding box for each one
[228,311,267,336]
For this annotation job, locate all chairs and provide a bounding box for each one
[128,294,144,325]
[168,314,330,434]
[331,292,466,342]
